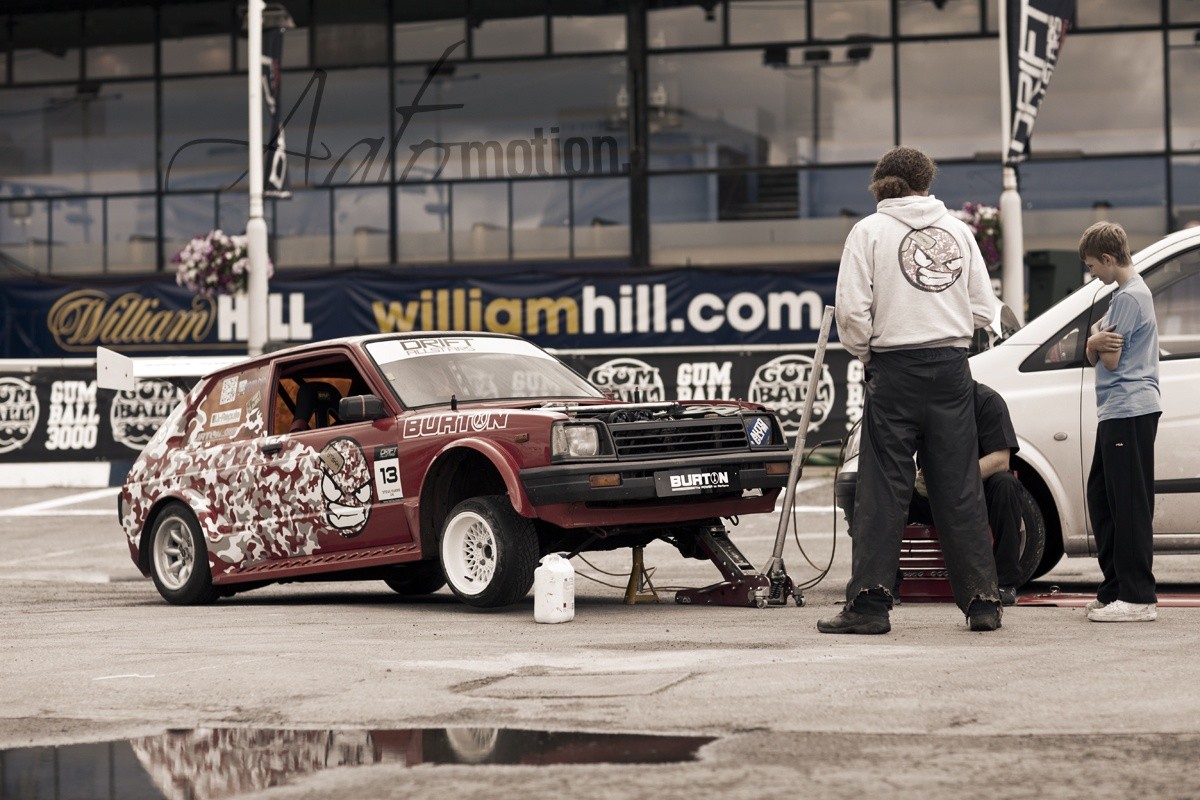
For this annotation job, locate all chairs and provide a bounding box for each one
[292,378,374,431]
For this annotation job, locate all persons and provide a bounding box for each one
[894,376,1023,607]
[1077,220,1164,621]
[816,147,1009,634]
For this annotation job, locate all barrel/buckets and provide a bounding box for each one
[533,554,575,624]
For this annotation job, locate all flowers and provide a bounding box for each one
[952,200,1004,271]
[169,230,273,297]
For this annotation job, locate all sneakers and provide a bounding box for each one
[970,601,1003,631]
[817,605,891,635]
[1086,600,1156,622]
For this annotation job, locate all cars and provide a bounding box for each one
[838,223,1200,596]
[114,331,801,608]
